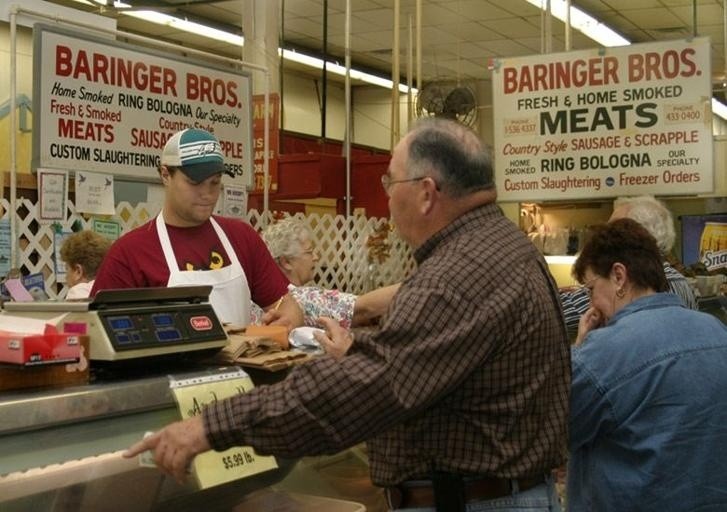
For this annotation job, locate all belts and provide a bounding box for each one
[381,471,543,511]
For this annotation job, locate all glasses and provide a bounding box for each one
[577,272,605,298]
[379,172,442,194]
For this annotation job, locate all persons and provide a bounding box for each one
[251,219,401,332]
[558,193,700,344]
[89,127,305,338]
[563,217,726,510]
[121,113,577,511]
[61,230,113,299]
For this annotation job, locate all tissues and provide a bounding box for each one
[1,304,82,368]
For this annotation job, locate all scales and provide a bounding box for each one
[1,285,231,361]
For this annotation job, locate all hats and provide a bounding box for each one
[159,126,234,182]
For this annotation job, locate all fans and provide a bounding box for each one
[413,71,484,131]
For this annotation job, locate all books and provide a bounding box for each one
[205,335,311,375]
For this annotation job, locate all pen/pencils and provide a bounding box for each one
[262,297,284,327]
